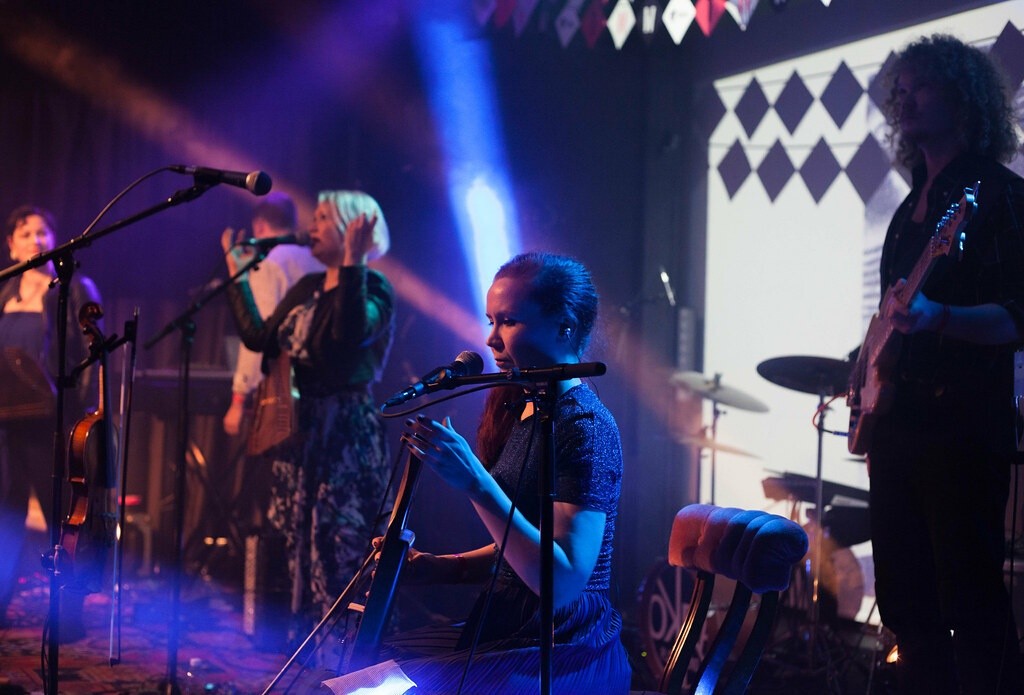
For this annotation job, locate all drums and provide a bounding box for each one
[637,558,703,682]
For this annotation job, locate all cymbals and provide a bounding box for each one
[670,370,769,418]
[756,356,852,395]
[678,433,757,463]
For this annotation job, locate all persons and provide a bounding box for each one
[0,204,105,644]
[222,191,394,665]
[848,33,1024,695]
[316,251,632,695]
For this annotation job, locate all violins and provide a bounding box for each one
[52,287,128,589]
[343,417,442,667]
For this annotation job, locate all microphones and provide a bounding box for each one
[242,229,312,248]
[170,165,272,195]
[658,264,676,307]
[383,351,483,407]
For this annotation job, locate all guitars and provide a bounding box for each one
[838,178,990,453]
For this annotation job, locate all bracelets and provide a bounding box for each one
[938,304,949,335]
